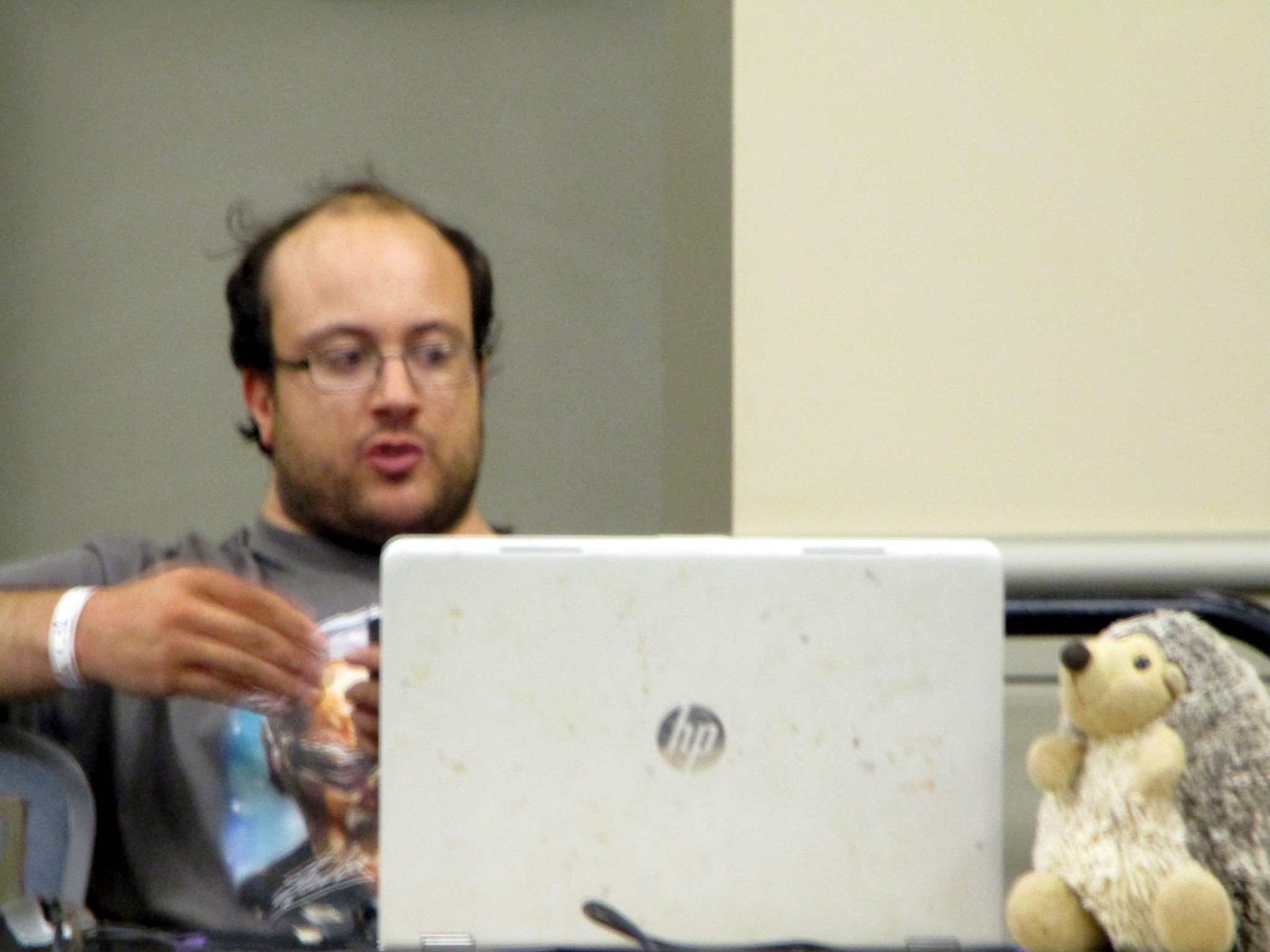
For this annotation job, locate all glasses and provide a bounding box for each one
[271,339,494,395]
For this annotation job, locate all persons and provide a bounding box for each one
[0,168,519,952]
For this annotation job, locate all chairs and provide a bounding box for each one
[1003,597,1269,883]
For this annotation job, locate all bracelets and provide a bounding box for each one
[46,585,108,690]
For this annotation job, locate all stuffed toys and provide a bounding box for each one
[1006,606,1270,952]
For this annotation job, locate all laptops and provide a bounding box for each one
[373,532,1005,952]
